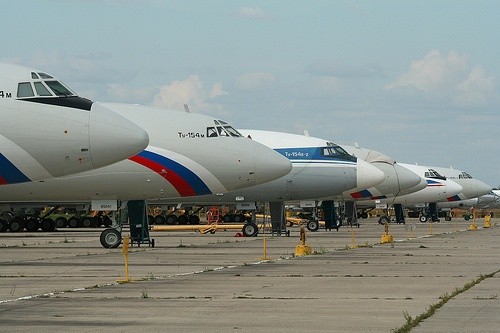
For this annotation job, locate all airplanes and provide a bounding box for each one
[303,140,500,239]
[0,64,150,184]
[1,98,293,249]
[146,126,383,239]
